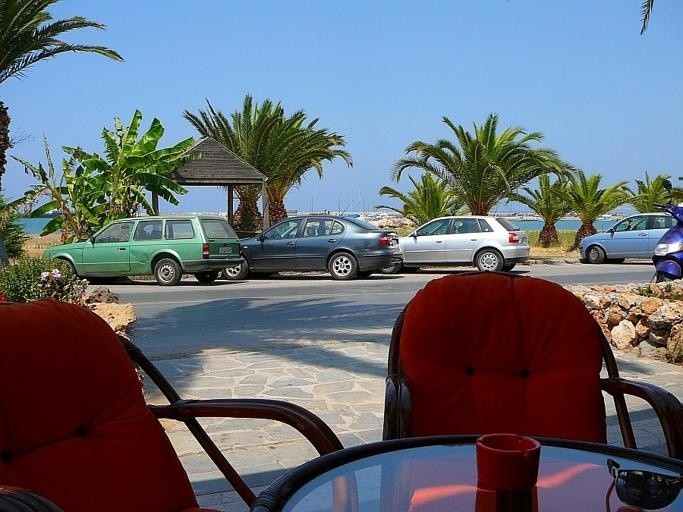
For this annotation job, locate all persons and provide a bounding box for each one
[119,224,129,241]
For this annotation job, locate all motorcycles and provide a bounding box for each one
[647,177,683,283]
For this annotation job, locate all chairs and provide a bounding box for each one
[381,270,683,512]
[0,299,358,512]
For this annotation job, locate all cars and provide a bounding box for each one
[221,212,403,280]
[38,211,245,290]
[573,208,680,266]
[379,213,534,275]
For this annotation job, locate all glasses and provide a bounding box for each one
[606,458,682,512]
